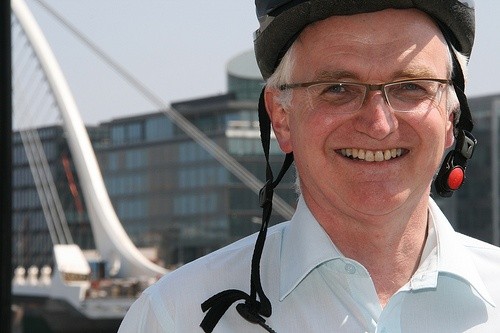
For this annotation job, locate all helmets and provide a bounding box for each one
[252,0,475,82]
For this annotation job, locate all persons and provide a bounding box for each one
[115,1,500,332]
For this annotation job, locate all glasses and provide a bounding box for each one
[269,77,455,115]
